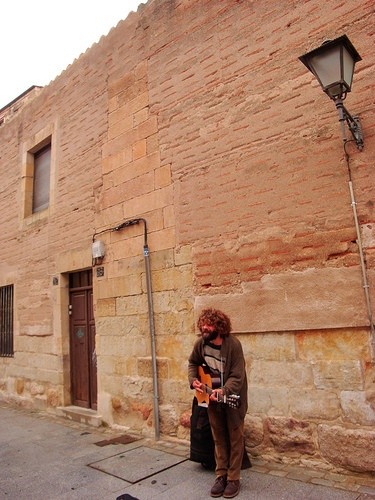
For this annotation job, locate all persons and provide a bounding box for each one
[187,307,248,498]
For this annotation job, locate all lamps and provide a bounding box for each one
[297,34,365,153]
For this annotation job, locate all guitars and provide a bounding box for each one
[194,365,241,411]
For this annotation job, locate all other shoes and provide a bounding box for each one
[210,475,226,497]
[223,479,240,498]
